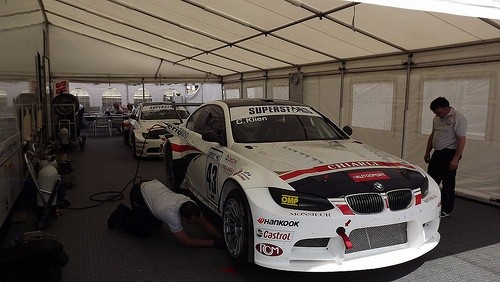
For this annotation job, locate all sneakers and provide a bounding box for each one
[440,209,452,218]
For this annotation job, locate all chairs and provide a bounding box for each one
[93,114,112,136]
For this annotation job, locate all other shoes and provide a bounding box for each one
[106,204,127,226]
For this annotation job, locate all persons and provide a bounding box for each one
[107,179,226,249]
[424,97,467,217]
[110,102,135,134]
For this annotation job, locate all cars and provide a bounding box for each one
[164,98,442,273]
[122,102,191,158]
